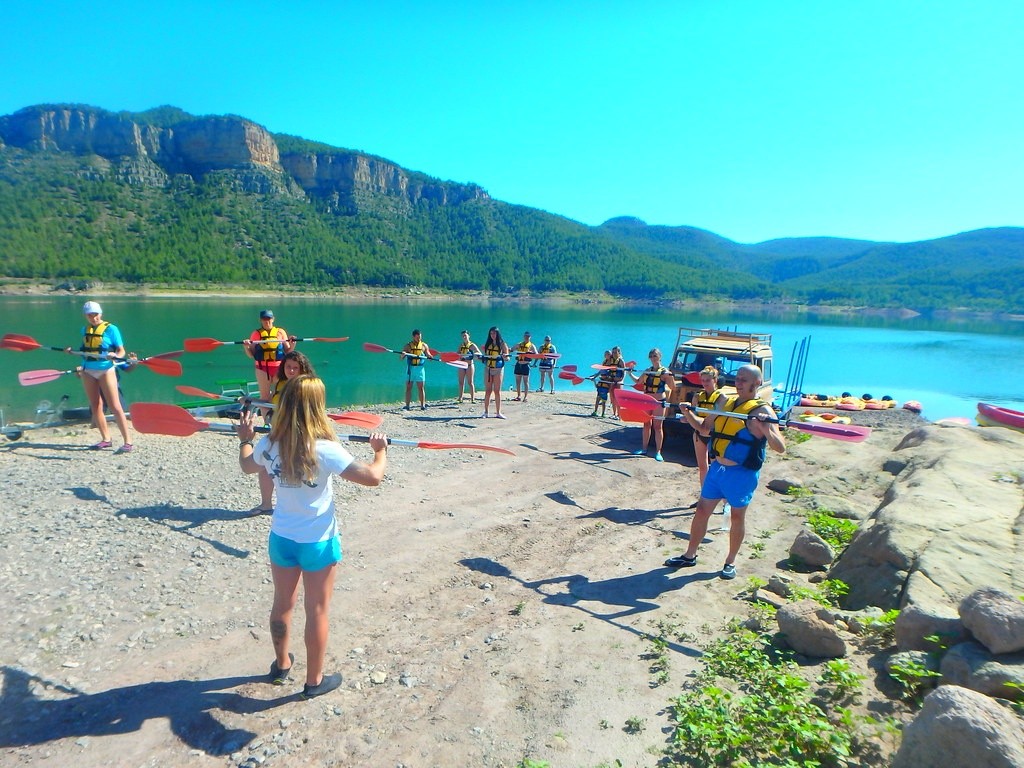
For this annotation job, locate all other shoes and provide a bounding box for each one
[471,400,476,404]
[722,563,736,579]
[460,399,463,403]
[601,413,606,418]
[536,388,544,392]
[633,448,648,454]
[421,406,427,410]
[482,412,488,418]
[495,413,505,419]
[613,416,620,420]
[656,452,663,460]
[550,390,555,394]
[300,673,342,699]
[608,415,616,418]
[264,423,270,429]
[523,397,527,402]
[270,654,294,684]
[93,439,113,450]
[403,406,411,410]
[665,555,699,566]
[121,443,134,451]
[591,411,599,416]
[514,397,521,401]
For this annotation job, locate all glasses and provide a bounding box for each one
[544,340,551,341]
[649,355,659,358]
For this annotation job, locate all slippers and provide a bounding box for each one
[249,505,273,517]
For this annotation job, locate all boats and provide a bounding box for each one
[975,412,1024,438]
[174,380,270,413]
[977,401,1024,429]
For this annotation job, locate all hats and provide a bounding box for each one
[524,331,531,336]
[84,301,104,314]
[259,309,274,319]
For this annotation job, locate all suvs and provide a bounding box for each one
[651,327,775,445]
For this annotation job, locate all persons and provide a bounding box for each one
[588,373,611,419]
[678,364,728,494]
[662,364,787,580]
[64,301,138,455]
[508,331,538,403]
[533,334,558,394]
[242,307,296,427]
[603,345,627,419]
[627,347,676,461]
[400,328,433,410]
[246,348,316,519]
[455,330,482,405]
[230,371,389,699]
[482,326,510,419]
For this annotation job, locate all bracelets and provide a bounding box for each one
[239,440,254,448]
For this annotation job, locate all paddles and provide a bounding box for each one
[129,401,517,459]
[440,352,546,363]
[428,348,441,356]
[18,350,184,387]
[1,334,183,377]
[184,336,350,353]
[616,408,681,427]
[512,365,578,372]
[481,345,561,359]
[590,363,702,386]
[614,386,872,444]
[363,343,469,369]
[175,384,383,431]
[558,360,646,392]
